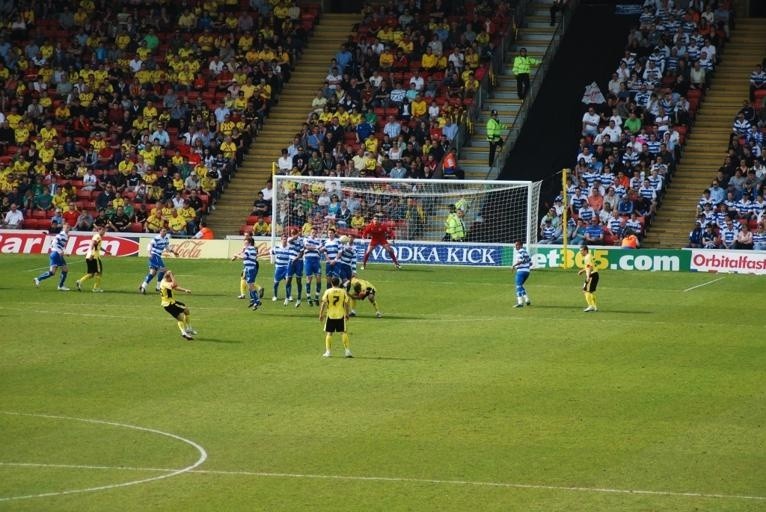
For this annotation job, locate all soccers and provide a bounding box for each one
[340,235,349,245]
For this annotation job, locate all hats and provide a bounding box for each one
[491,108,498,116]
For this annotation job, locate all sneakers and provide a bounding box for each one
[155,287,160,291]
[348,311,356,316]
[75,280,82,292]
[323,351,331,357]
[375,312,383,318]
[344,349,353,357]
[56,285,70,291]
[359,265,403,271]
[512,299,531,308]
[33,277,41,287]
[91,287,104,293]
[181,328,198,341]
[583,306,597,313]
[270,296,320,309]
[139,286,146,295]
[237,287,264,311]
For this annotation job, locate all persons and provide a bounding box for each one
[159,270,199,340]
[230,227,358,311]
[138,228,178,295]
[577,245,599,313]
[75,227,112,292]
[317,280,351,357]
[351,276,382,318]
[0,1,766,250]
[510,241,532,307]
[31,223,71,291]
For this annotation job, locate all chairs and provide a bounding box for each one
[754,89,766,109]
[687,89,701,116]
[673,126,686,146]
